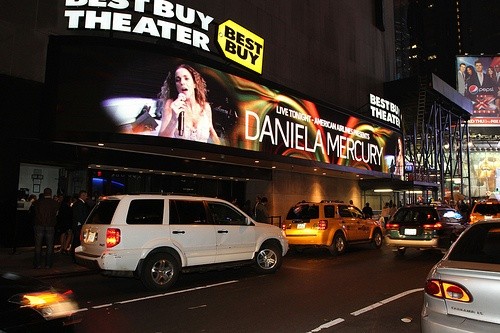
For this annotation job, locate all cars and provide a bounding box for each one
[422,220,499,333]
[467,197,500,226]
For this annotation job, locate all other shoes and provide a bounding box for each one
[45,264,51,269]
[33,264,41,269]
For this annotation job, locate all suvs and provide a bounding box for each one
[74,196,290,294]
[384,202,470,256]
[284,200,384,253]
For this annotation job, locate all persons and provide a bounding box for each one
[428,197,489,226]
[458,61,500,118]
[394,136,404,175]
[155,64,221,145]
[302,200,402,233]
[29,188,124,268]
[216,195,271,224]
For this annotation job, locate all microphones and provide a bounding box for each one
[178,93,186,136]
